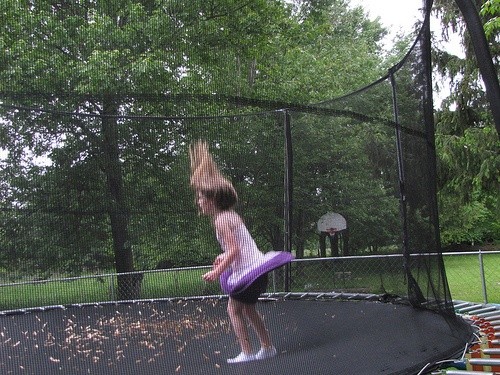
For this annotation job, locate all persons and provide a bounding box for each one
[187,138,277,365]
